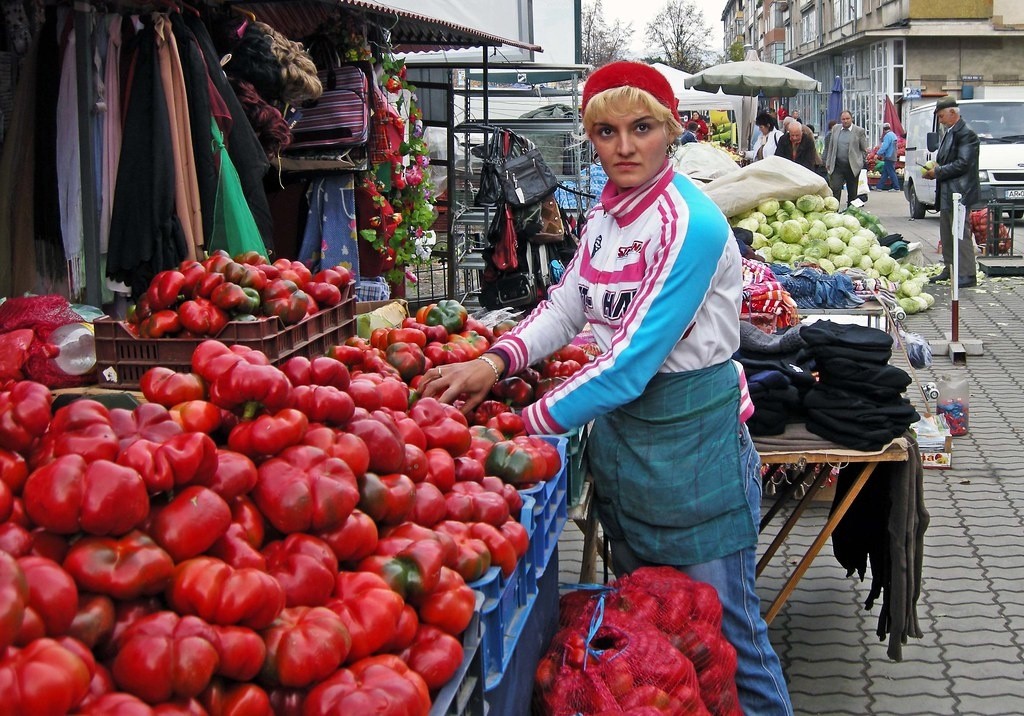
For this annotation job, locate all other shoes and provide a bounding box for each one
[872,185,882,192]
[888,188,901,192]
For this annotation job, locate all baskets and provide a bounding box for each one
[434,400,593,715]
[92,278,358,388]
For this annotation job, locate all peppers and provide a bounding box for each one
[0,296,603,716]
[535,565,742,716]
[123,250,352,338]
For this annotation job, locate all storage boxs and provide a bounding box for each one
[428,417,588,716]
[867,177,892,184]
[36,298,411,408]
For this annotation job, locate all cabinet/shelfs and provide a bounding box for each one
[440,63,593,315]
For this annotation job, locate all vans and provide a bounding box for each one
[904,97,1024,227]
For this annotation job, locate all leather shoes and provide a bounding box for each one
[930,269,950,283]
[958,275,978,288]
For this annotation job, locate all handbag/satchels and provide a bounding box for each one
[267,36,390,171]
[472,127,565,317]
[857,169,871,196]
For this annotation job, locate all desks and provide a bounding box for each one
[786,298,884,330]
[739,320,908,625]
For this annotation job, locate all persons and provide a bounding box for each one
[750,104,837,185]
[871,123,901,192]
[680,112,708,145]
[416,61,794,715]
[922,96,981,287]
[825,111,868,213]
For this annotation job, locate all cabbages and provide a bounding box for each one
[920,161,933,175]
[730,192,936,314]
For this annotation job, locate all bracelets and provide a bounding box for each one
[478,356,499,388]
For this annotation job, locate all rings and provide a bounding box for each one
[438,368,442,375]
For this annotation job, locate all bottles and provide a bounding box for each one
[935,369,970,436]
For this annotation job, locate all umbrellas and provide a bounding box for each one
[825,76,844,135]
[684,61,822,150]
[879,94,905,140]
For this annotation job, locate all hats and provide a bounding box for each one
[582,62,681,121]
[884,123,890,127]
[766,260,865,310]
[934,95,959,114]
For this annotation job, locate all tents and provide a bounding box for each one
[570,61,760,154]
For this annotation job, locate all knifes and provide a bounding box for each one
[916,162,931,171]
